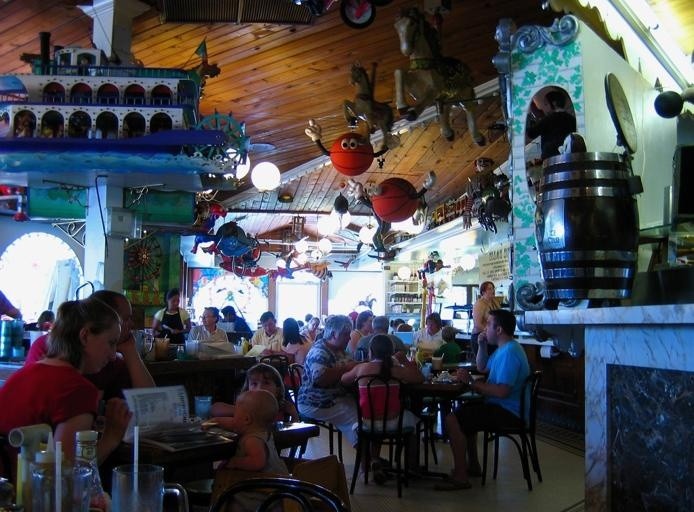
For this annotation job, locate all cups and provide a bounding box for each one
[194,396,211,419]
[30,465,94,511]
[154,337,168,360]
[425,362,432,372]
[110,464,189,512]
[185,339,198,355]
[12,346,25,358]
[271,341,279,352]
[431,356,442,370]
[422,368,430,381]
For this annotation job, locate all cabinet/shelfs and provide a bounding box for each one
[386,276,424,308]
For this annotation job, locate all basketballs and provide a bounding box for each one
[370,178,419,223]
[329,132,374,176]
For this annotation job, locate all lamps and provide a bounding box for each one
[218,148,249,190]
[249,158,284,213]
[272,203,430,271]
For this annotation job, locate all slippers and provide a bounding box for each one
[435,477,470,491]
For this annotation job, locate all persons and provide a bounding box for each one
[201,363,300,512]
[1,291,157,512]
[297,281,533,492]
[156,287,322,405]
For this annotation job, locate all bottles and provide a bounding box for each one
[391,294,422,302]
[71,430,106,511]
[33,429,67,510]
[143,329,154,352]
[240,337,253,353]
[176,345,184,359]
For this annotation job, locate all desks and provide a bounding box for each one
[412,372,486,473]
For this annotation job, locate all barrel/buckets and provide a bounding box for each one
[536,72,643,311]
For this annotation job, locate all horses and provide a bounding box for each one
[353,298,377,311]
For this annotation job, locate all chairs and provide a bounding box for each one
[347,372,412,500]
[0,313,346,512]
[480,368,545,494]
